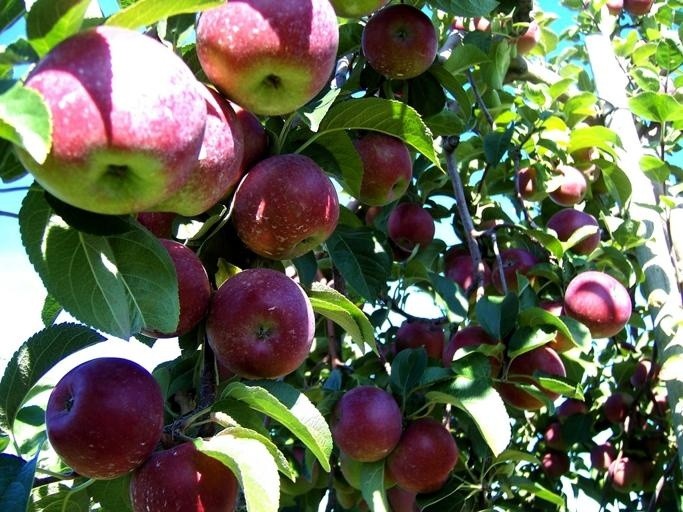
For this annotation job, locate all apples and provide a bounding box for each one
[15,1,669,512]
